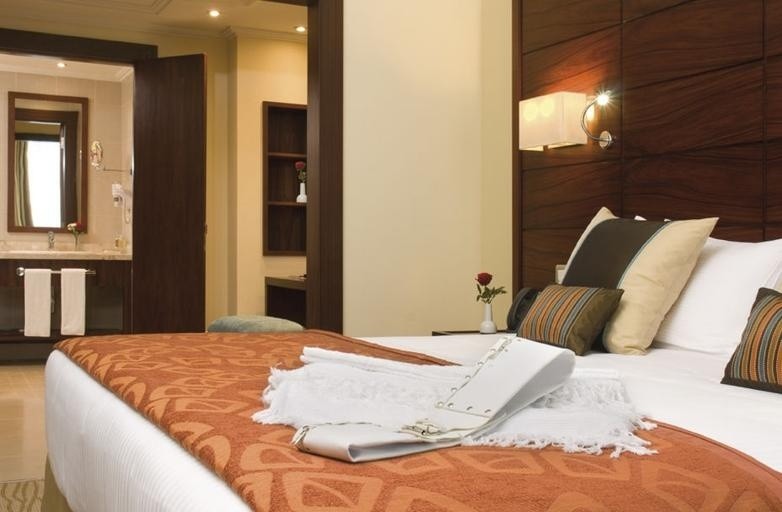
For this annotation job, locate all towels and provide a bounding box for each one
[22,268,52,337]
[60,268,86,336]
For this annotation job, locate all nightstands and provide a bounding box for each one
[431,328,518,336]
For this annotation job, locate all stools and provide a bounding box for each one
[207,313,304,334]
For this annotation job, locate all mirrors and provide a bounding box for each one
[88,138,132,176]
[7,92,89,232]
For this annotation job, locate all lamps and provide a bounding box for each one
[516,91,589,152]
[581,87,615,151]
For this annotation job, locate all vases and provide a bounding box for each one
[72,234,80,251]
[296,183,307,203]
[479,302,497,334]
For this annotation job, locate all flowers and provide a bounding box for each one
[66,222,84,246]
[295,161,307,183]
[473,272,507,303]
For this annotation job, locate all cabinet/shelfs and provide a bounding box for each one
[262,101,307,257]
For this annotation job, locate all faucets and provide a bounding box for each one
[47,231,55,251]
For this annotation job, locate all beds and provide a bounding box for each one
[42,331,782,512]
